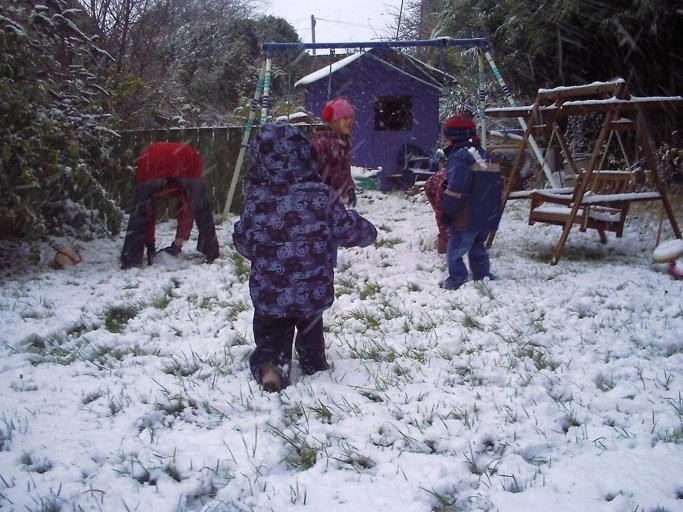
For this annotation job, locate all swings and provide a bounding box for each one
[397,47,451,175]
[529,95,636,239]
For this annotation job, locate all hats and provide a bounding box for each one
[323,98,354,125]
[443,108,476,142]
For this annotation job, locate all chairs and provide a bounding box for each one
[526,168,643,238]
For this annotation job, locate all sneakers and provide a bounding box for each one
[261,367,283,391]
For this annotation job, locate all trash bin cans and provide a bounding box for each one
[538,147,555,174]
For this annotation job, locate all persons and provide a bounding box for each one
[438,115,503,293]
[311,98,358,268]
[119,142,219,269]
[231,124,377,391]
[424,148,454,254]
